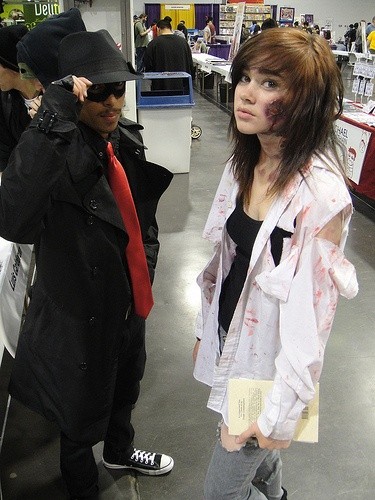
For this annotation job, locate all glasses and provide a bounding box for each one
[86,82,126,102]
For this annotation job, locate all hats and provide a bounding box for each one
[0,25,29,74]
[53,29,145,84]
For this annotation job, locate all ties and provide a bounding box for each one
[106,141,154,319]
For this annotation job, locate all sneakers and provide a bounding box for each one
[102,448,174,475]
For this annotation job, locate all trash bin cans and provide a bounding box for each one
[137,72,195,173]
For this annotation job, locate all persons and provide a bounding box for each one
[344,16,375,54]
[277,20,333,44]
[203,19,213,44]
[240,21,262,44]
[0,29,175,499]
[134,14,151,72]
[142,19,194,91]
[0,24,43,174]
[148,16,188,46]
[192,27,359,500]
[207,16,216,44]
[192,35,207,53]
[261,18,279,31]
[17,8,87,94]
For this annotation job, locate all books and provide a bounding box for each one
[227,36,233,43]
[243,13,271,20]
[244,21,263,27]
[220,5,239,12]
[229,377,320,443]
[220,13,237,20]
[220,21,235,27]
[220,27,234,34]
[244,5,271,13]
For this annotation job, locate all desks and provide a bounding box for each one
[192,53,375,211]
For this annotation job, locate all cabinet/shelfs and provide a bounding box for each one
[219,6,272,36]
